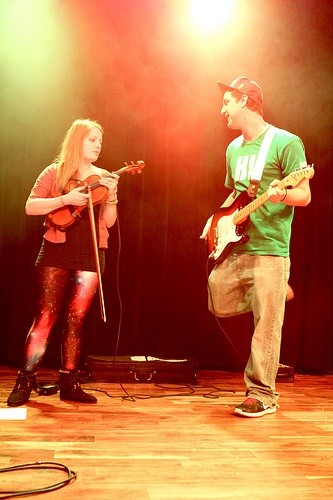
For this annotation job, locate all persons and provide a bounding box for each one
[200,76,312,418]
[7,118,117,407]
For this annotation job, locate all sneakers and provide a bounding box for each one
[233,396,278,418]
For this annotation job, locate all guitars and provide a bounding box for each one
[207,163,315,264]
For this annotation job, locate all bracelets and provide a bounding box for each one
[60,196,65,206]
[105,193,118,205]
[280,189,287,202]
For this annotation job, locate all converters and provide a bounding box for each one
[39,386,57,396]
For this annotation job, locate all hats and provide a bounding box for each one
[217,76,264,109]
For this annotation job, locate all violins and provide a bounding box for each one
[44,160,146,228]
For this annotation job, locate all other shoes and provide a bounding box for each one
[8,371,38,406]
[59,369,98,403]
